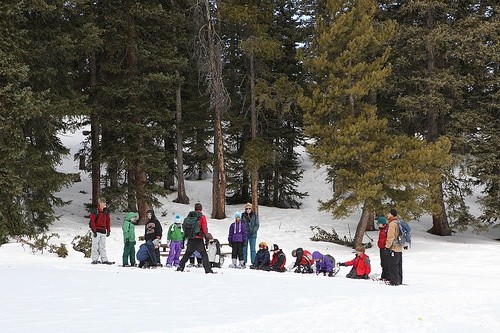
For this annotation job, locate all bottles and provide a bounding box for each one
[391,250,395,256]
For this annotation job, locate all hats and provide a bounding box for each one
[259,242,266,248]
[378,217,386,225]
[152,239,160,247]
[171,215,184,232]
[234,212,242,233]
[195,203,202,211]
[390,210,397,216]
[98,197,108,202]
[270,245,278,251]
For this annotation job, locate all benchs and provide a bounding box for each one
[159,244,220,268]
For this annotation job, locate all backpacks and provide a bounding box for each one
[182,211,202,239]
[394,219,411,249]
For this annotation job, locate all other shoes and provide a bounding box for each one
[93,260,97,263]
[131,264,137,266]
[124,264,130,266]
[103,261,111,264]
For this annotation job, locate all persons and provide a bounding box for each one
[90,197,111,264]
[268,244,286,272]
[187,250,203,267]
[251,242,270,270]
[145,209,163,265]
[377,217,388,281]
[384,209,403,286]
[228,212,248,269]
[177,203,213,274]
[122,212,137,267]
[311,251,333,277]
[241,203,259,266]
[339,246,371,279]
[137,238,161,269]
[205,233,220,266]
[292,248,314,273]
[166,214,185,266]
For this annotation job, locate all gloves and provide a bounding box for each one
[126,238,129,245]
[242,240,246,246]
[94,232,97,237]
[229,241,232,247]
[363,274,368,278]
[339,263,345,265]
[384,248,389,255]
[167,240,171,247]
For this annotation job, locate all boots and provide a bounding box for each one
[237,260,244,268]
[229,258,236,268]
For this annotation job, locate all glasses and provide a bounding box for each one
[246,208,251,210]
